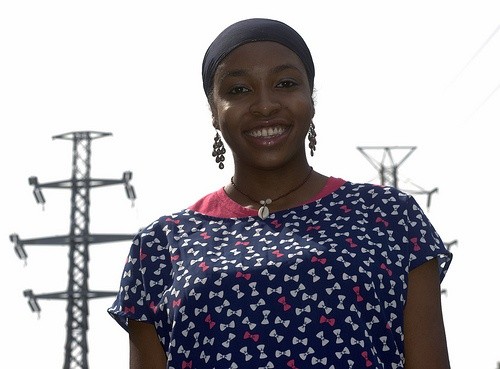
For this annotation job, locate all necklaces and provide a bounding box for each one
[231,166,314,220]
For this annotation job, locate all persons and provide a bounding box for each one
[106,18,453,369]
